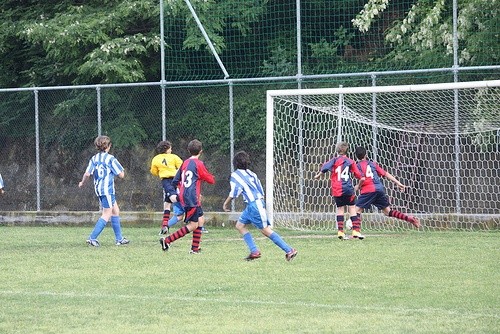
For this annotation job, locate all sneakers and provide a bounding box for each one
[86,237,100,247]
[190,248,201,255]
[246,250,261,261]
[159,237,170,251]
[286,247,298,261]
[160,227,169,234]
[115,237,130,245]
[201,227,208,234]
[408,216,420,228]
[338,231,345,239]
[352,230,364,240]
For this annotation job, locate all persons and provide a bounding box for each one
[222,150,298,261]
[314,142,365,240]
[150,140,184,235]
[0,174,5,197]
[159,139,215,255]
[346,147,421,230]
[78,136,130,247]
